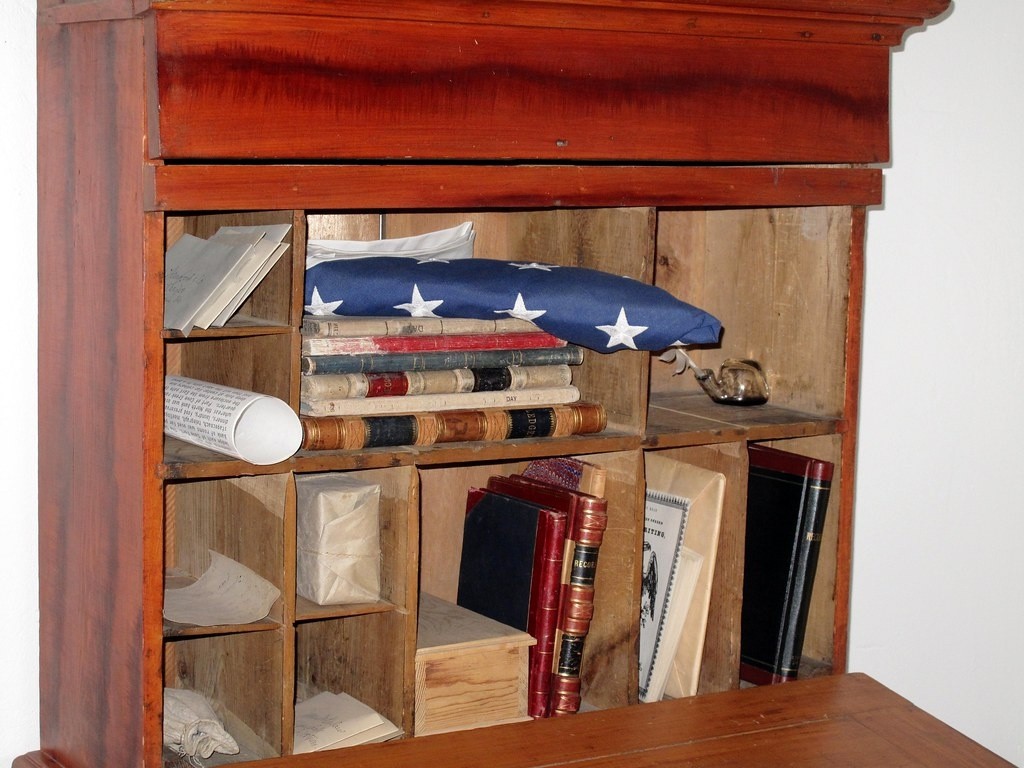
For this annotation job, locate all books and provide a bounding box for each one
[739,442,834,687]
[299,314,608,451]
[457,457,609,718]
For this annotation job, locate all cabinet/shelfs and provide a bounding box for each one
[36,2,953,767]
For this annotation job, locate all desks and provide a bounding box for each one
[184,673,1018,768]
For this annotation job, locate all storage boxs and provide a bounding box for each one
[414,591,537,738]
[297,481,382,605]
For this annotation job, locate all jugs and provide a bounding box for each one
[693,358,769,406]
